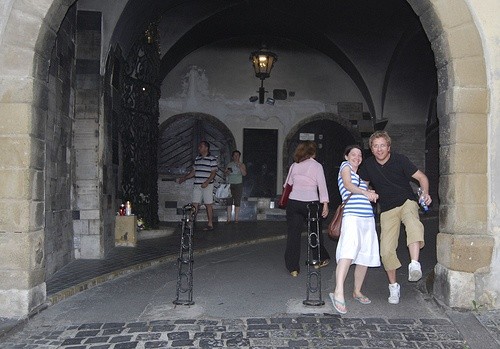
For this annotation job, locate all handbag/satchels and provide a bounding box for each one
[278,162,295,209]
[216,183,232,198]
[327,204,344,240]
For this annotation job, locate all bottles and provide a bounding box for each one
[119,204,126,216]
[125,201,131,215]
[417,187,428,210]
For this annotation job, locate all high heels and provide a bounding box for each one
[314,258,329,269]
[290,270,299,277]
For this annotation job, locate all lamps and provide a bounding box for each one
[249,44,278,104]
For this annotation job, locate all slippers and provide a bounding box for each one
[352,292,372,305]
[202,226,214,231]
[329,292,347,314]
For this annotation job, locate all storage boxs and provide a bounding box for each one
[114,215,138,248]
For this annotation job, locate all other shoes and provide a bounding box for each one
[225,221,232,225]
[234,221,239,224]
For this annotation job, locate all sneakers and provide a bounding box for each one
[408,259,422,281]
[388,282,401,304]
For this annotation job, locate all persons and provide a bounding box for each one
[224,150,247,226]
[356,130,432,305]
[182,140,218,232]
[279,141,331,277]
[329,145,382,314]
